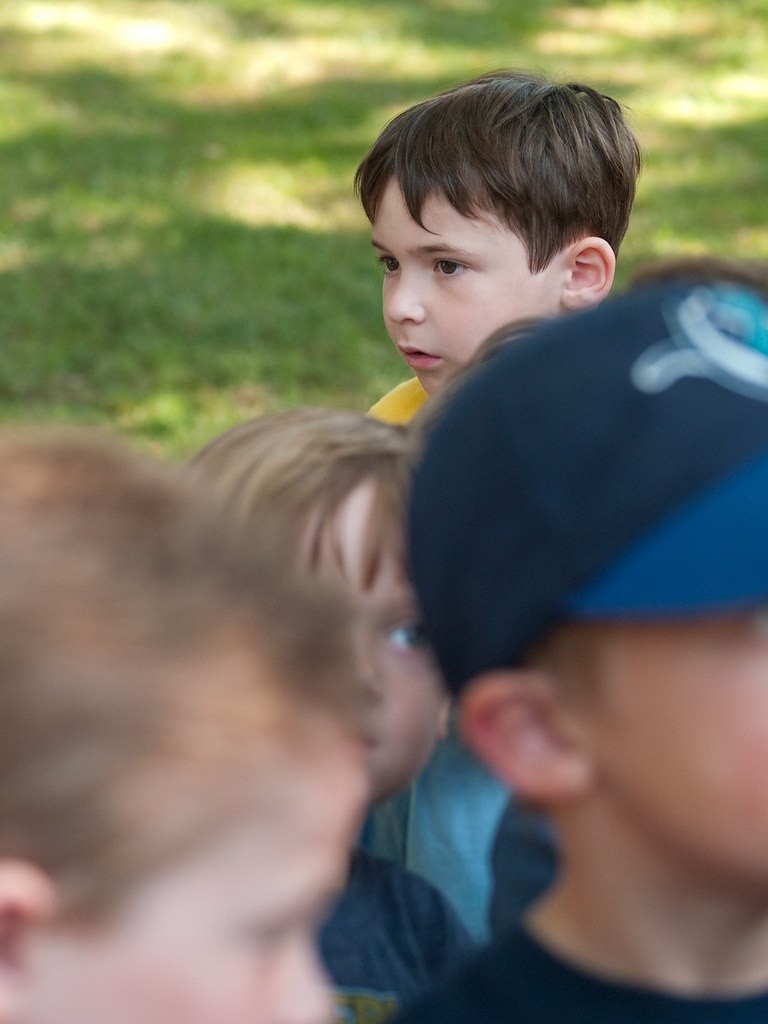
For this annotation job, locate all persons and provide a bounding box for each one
[404,258,767,1024]
[1,425,377,1023]
[181,404,485,1023]
[361,76,639,436]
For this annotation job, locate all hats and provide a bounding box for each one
[408,280,768,691]
